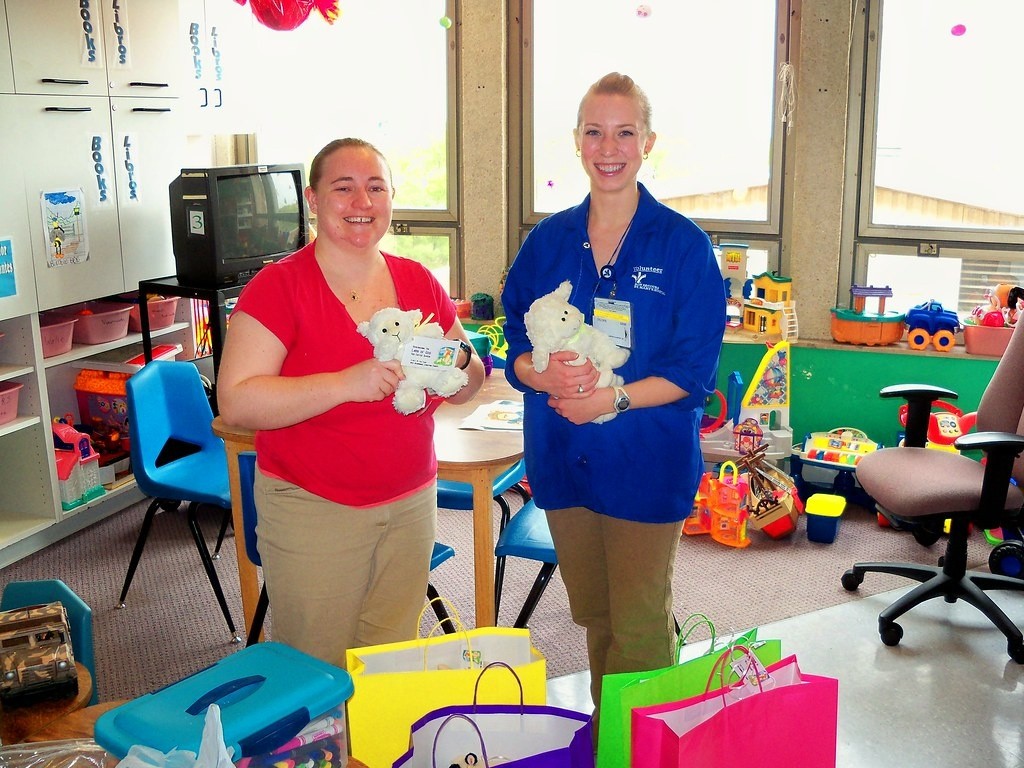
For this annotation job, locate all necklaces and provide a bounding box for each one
[349,289,360,301]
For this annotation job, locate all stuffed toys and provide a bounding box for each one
[521,275,631,389]
[356,306,469,416]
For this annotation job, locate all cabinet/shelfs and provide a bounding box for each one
[0,0,242,572]
[137,275,247,513]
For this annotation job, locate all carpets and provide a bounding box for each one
[0,494,991,699]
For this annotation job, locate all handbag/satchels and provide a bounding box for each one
[345,597,839,768]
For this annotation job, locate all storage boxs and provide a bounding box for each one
[805,493,847,544]
[92,640,355,768]
[959,320,1015,357]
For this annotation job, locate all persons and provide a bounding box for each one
[214,135,487,673]
[499,73,727,768]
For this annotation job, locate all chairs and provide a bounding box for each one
[841,309,1024,666]
[1,580,99,707]
[115,360,686,645]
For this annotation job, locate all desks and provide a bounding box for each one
[1,661,368,768]
[214,367,523,642]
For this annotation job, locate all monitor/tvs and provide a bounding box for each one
[169,163,310,290]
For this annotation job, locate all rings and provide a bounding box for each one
[577,384,584,393]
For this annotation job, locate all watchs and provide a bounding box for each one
[453,339,472,370]
[610,383,631,414]
